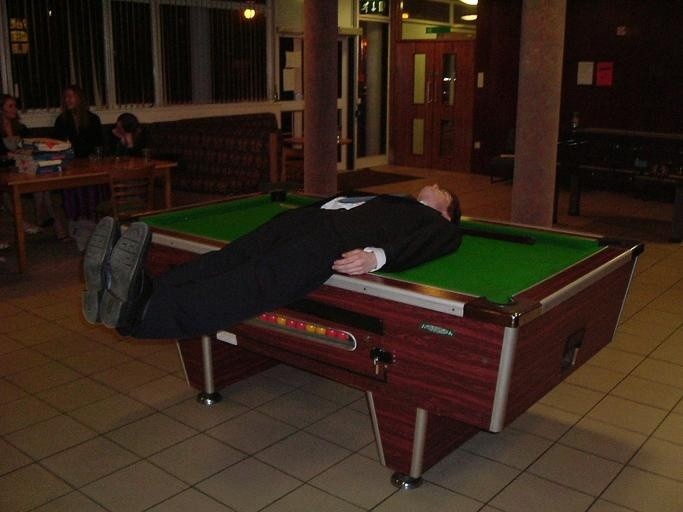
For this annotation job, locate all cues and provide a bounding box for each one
[278,202,538,247]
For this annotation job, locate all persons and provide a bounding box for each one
[54,85,105,253]
[108,112,149,245]
[80,182,464,340]
[1,92,56,236]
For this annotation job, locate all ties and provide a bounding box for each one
[338,193,416,203]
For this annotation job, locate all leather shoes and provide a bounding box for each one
[80,216,121,324]
[99,222,159,336]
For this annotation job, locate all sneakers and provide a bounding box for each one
[23,222,41,235]
[0,239,10,250]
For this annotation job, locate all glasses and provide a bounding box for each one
[439,188,451,199]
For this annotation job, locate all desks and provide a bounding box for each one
[284,138,353,176]
[114,190,644,490]
[0,156,178,273]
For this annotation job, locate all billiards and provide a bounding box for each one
[258,313,350,344]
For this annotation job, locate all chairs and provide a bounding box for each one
[107,164,155,216]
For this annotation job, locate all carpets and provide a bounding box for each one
[339,167,424,188]
